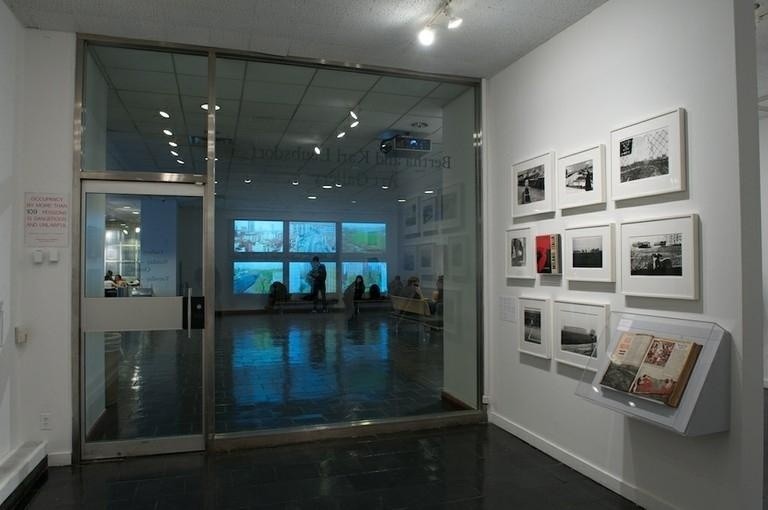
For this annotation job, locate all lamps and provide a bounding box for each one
[158,103,435,202]
[417,0,464,47]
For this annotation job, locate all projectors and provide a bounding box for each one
[380,135,432,159]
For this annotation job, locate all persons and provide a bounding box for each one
[103,270,127,289]
[388,275,403,298]
[307,256,330,314]
[430,274,442,314]
[519,177,534,204]
[401,277,433,319]
[346,275,365,301]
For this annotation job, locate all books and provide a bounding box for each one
[598,329,704,409]
[535,233,562,277]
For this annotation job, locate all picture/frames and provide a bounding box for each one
[557,144,606,209]
[610,107,687,202]
[397,183,462,287]
[619,213,700,301]
[518,296,552,360]
[563,223,617,283]
[553,299,610,372]
[503,227,535,279]
[510,151,555,218]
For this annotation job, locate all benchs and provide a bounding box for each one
[354,298,393,312]
[275,299,338,312]
[389,295,444,344]
